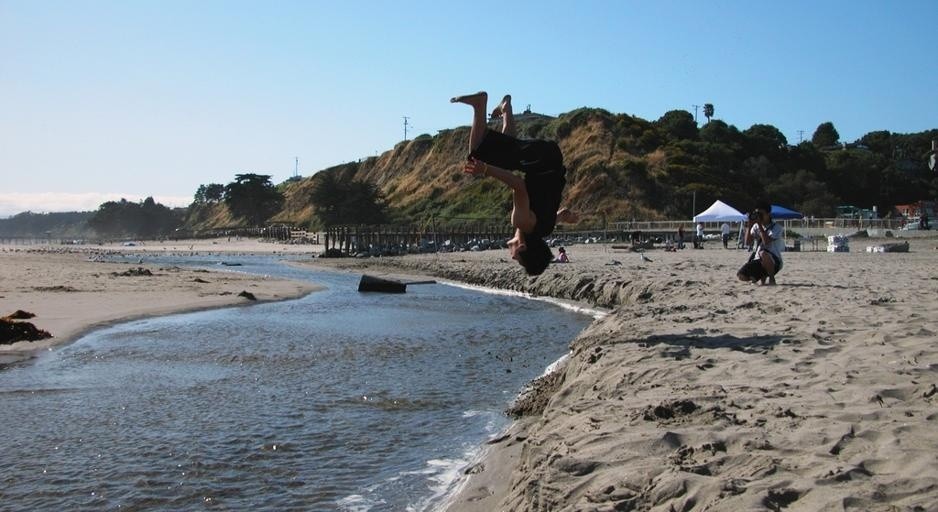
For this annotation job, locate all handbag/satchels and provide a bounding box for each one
[748,250,756,260]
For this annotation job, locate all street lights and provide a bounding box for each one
[686,188,696,216]
[872,205,877,227]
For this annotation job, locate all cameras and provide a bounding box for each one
[748,212,761,221]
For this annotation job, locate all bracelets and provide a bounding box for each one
[483,162,488,174]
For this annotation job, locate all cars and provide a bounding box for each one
[260,223,298,238]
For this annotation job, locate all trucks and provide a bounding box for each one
[897,216,933,231]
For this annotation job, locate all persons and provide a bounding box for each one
[450,91,566,277]
[919,210,929,229]
[678,222,685,249]
[800,213,817,228]
[696,222,704,249]
[867,214,875,228]
[721,222,730,249]
[737,201,784,286]
[858,214,863,230]
[553,246,568,263]
